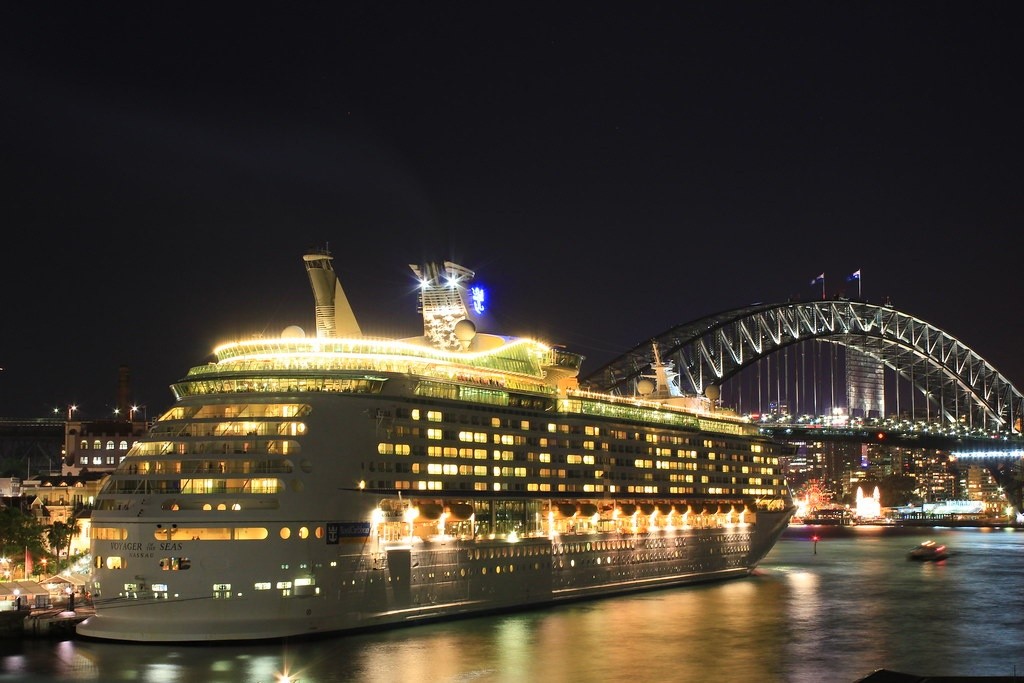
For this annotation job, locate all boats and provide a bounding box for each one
[691,503,705,515]
[639,503,653,514]
[801,508,848,526]
[619,502,637,517]
[719,503,732,515]
[378,497,407,523]
[556,503,576,517]
[733,503,745,513]
[580,504,598,518]
[705,504,719,514]
[659,502,673,515]
[418,501,444,521]
[450,503,474,520]
[906,539,946,561]
[674,502,688,514]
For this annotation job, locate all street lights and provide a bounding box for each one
[68,405,77,420]
[129,406,138,422]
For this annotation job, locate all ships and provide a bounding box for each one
[73,253,799,648]
